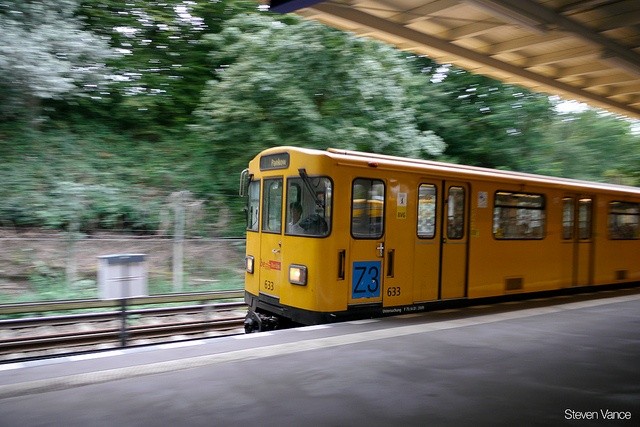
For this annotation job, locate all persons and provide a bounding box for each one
[286,202,305,234]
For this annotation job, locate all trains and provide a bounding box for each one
[239,145,640,335]
